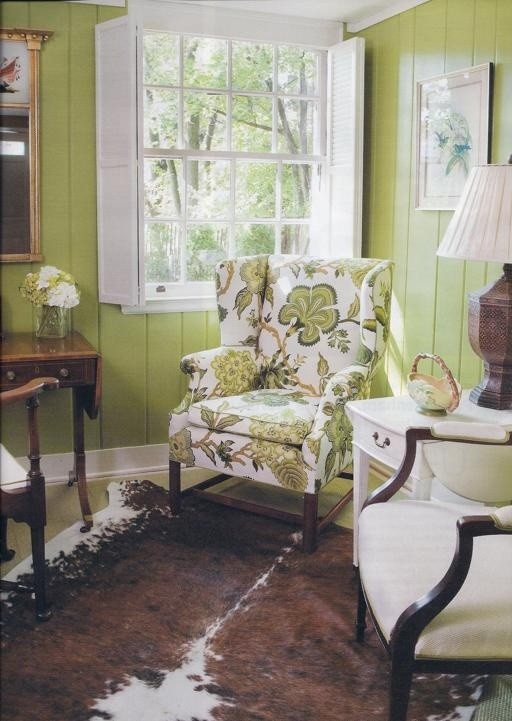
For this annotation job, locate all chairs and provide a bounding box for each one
[0,377,60,620]
[356,421,512,720]
[168,255,395,554]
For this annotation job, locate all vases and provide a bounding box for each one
[34,306,72,340]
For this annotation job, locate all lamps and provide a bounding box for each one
[436,154,512,410]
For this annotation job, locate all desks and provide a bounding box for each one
[344,390,512,568]
[0,333,102,527]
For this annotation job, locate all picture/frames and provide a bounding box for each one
[413,62,493,211]
[0,28,53,263]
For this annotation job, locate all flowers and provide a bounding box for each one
[18,266,81,333]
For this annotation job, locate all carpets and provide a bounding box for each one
[0,479,487,721]
